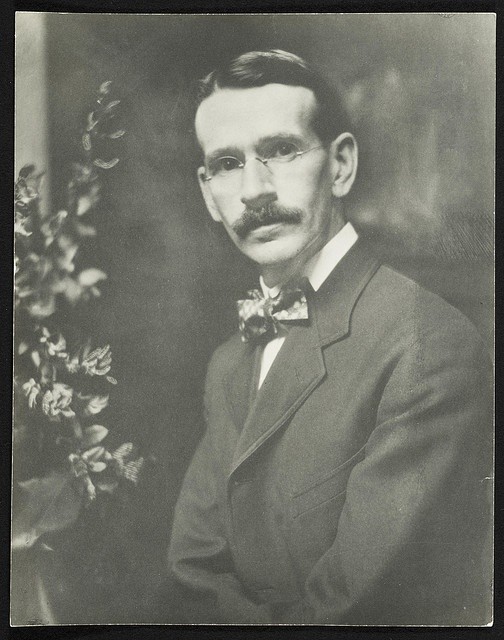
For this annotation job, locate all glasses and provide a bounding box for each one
[205,140,327,182]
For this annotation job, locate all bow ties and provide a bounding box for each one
[235,280,309,343]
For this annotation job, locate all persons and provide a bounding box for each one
[163,47,493,626]
